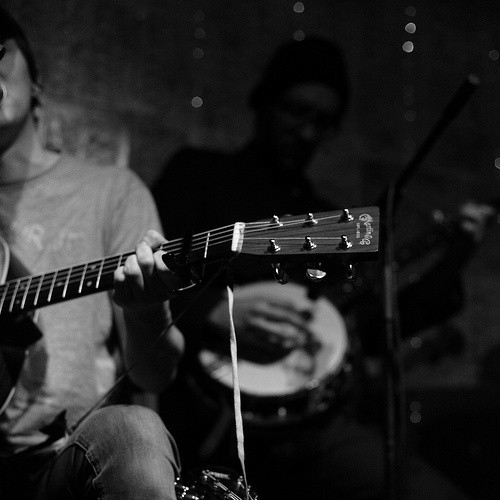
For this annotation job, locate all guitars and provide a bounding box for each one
[0,205,381,414]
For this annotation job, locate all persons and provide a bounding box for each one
[1,16,183,500]
[151,35,489,475]
